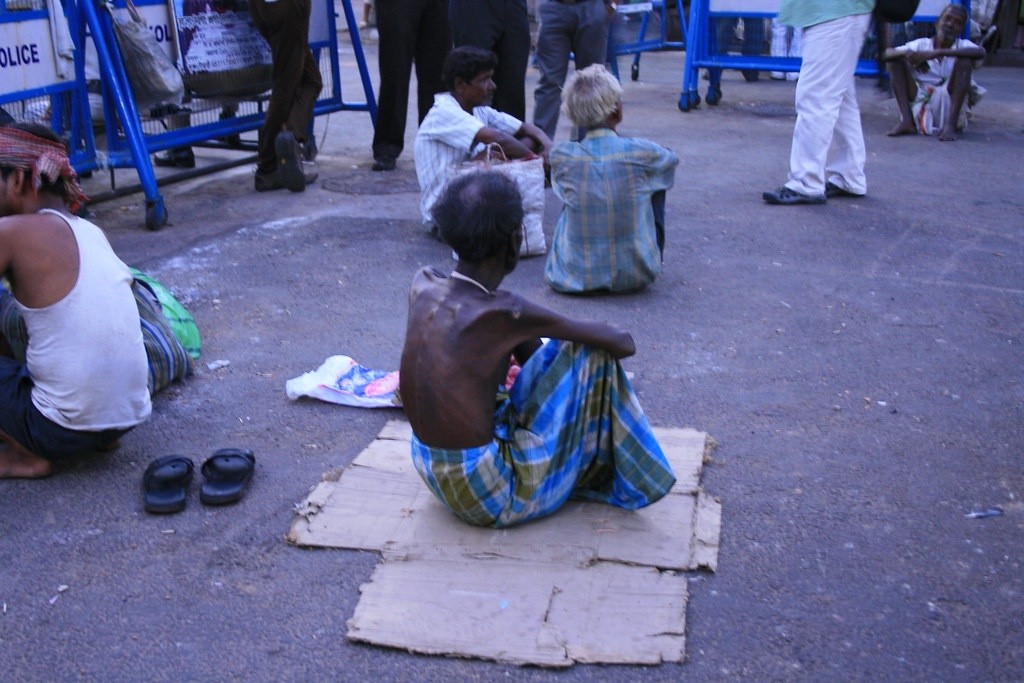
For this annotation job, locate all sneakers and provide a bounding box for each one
[825,183,864,197]
[763,188,827,205]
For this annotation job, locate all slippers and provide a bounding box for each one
[144,456,193,511]
[200,445,255,503]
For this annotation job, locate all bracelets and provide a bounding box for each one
[607,0,616,11]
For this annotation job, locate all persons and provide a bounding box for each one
[762,0,876,205]
[358,0,377,28]
[1014,0,1024,49]
[400,169,676,531]
[153,104,242,168]
[545,63,678,293]
[247,0,322,191]
[0,123,153,477]
[414,46,553,238]
[446,0,532,124]
[883,5,986,141]
[702,18,801,81]
[373,0,445,169]
[534,0,621,141]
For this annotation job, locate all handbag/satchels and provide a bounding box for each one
[177,0,272,94]
[286,354,403,408]
[0,265,201,400]
[447,143,546,256]
[104,0,184,108]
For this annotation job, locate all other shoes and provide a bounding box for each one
[275,132,306,193]
[254,164,317,192]
[154,149,196,168]
[372,160,396,171]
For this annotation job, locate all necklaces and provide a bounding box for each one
[451,271,489,293]
